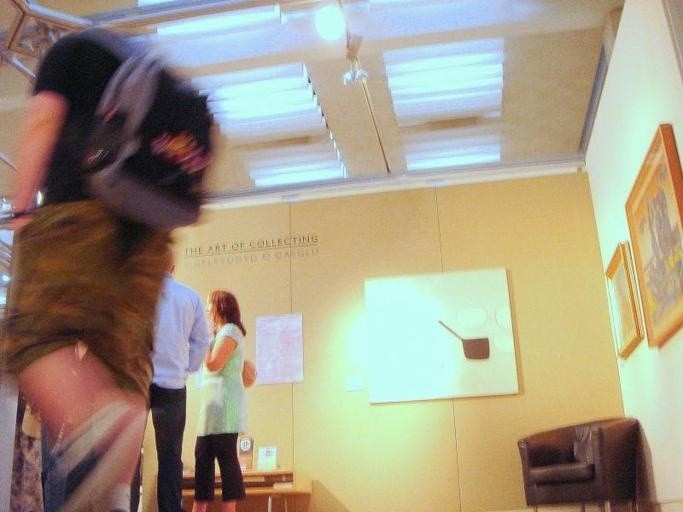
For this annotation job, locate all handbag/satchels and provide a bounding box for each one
[242,360,257,388]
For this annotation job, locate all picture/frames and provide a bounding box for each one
[606,242,644,358]
[625,122,683,348]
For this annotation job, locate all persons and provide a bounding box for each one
[126,243,210,509]
[2,22,176,511]
[188,287,250,511]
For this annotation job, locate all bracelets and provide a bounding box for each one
[11,208,41,221]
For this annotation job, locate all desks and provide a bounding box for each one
[182,471,312,511]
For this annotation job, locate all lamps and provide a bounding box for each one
[343,54,367,87]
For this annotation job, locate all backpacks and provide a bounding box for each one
[79,27,214,227]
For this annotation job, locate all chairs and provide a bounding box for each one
[518,417,638,512]
